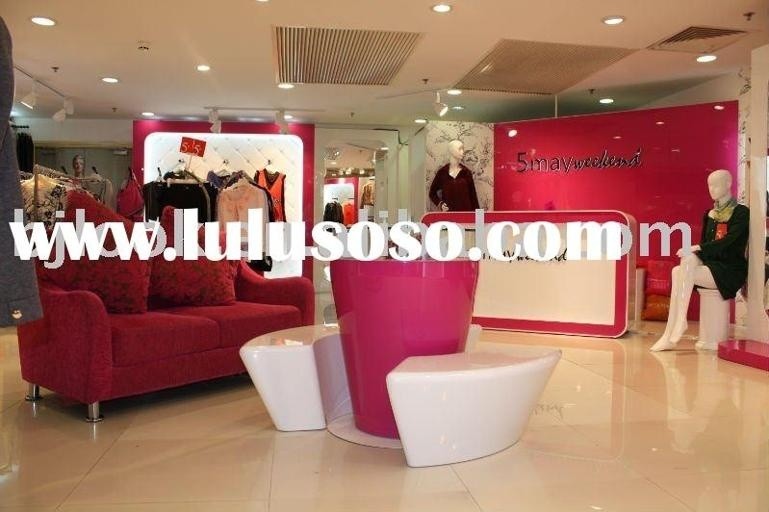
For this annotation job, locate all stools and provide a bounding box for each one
[694,288,736,353]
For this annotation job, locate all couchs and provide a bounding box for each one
[17,190,315,424]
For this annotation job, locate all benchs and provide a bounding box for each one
[385,348,563,466]
[239,323,483,432]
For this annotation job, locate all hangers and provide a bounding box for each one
[24,163,291,185]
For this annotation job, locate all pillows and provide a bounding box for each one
[151,202,242,307]
[35,191,151,316]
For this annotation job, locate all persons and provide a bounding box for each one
[427,140,481,212]
[651,168,751,355]
[73,155,88,179]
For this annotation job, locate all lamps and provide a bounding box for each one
[20,78,69,123]
[431,90,449,117]
[209,111,288,136]
